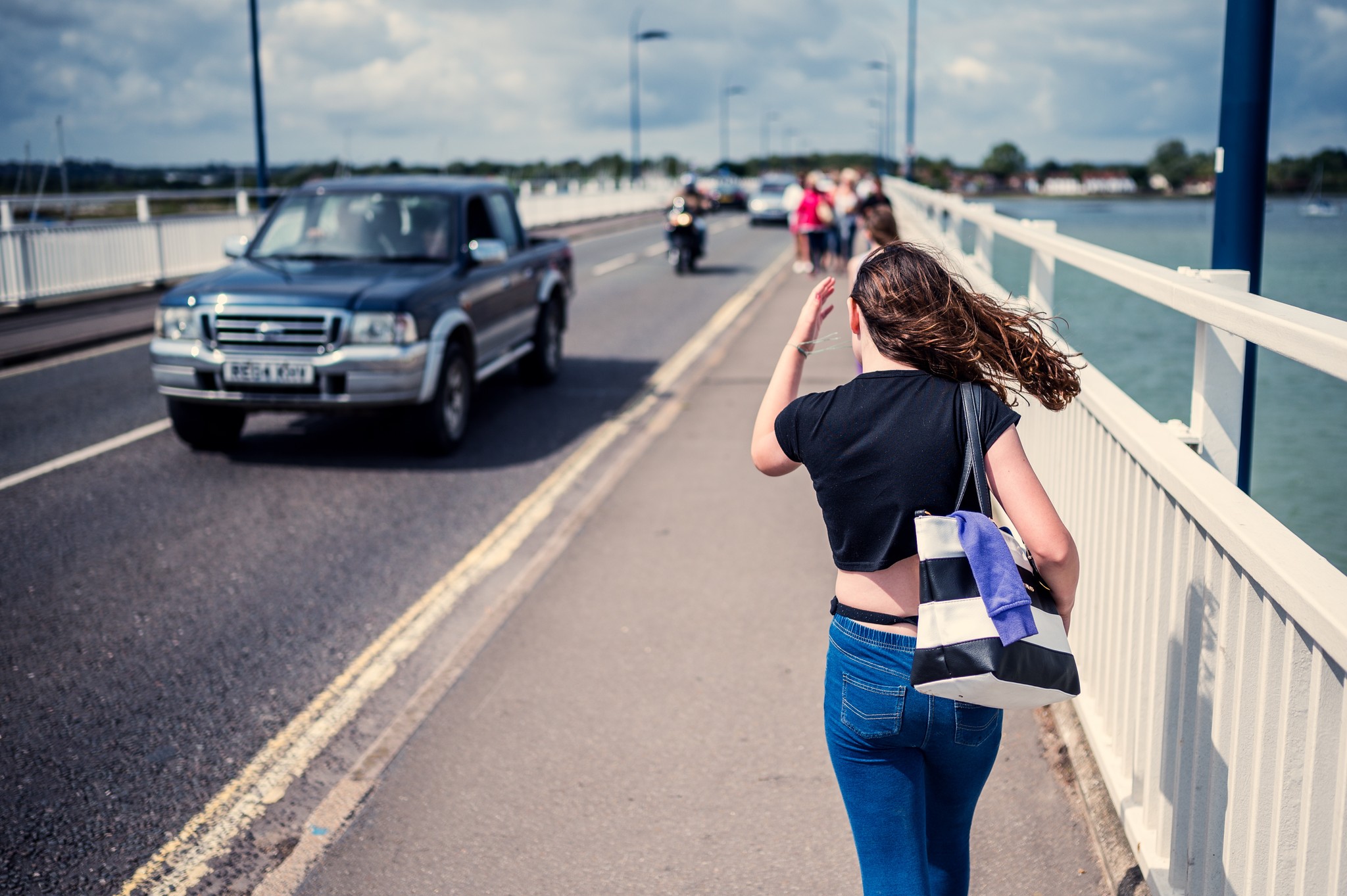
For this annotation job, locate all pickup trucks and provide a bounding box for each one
[147,175,577,454]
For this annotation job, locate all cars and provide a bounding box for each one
[716,184,745,205]
[747,172,799,224]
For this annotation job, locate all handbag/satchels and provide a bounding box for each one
[910,381,1081,712]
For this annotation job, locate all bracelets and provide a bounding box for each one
[787,341,808,357]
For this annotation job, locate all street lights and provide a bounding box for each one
[630,25,672,161]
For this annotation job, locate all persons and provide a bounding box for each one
[780,167,886,274]
[750,240,1082,896]
[664,173,715,260]
[305,194,384,260]
[846,193,902,375]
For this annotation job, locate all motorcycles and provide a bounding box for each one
[661,199,706,274]
